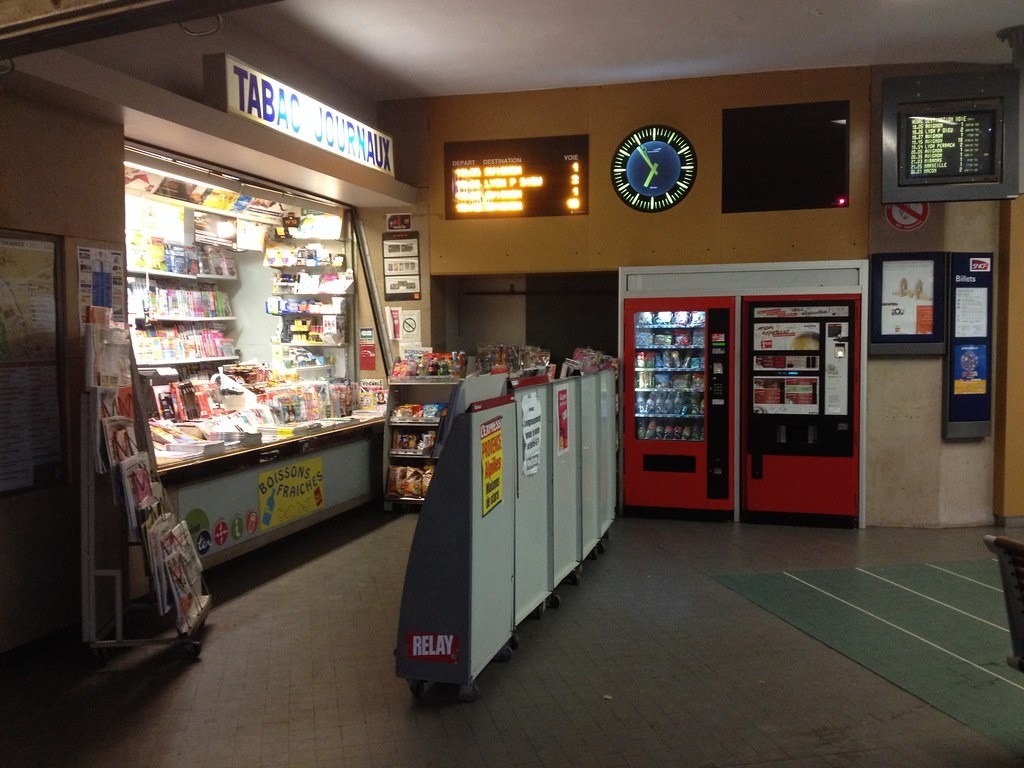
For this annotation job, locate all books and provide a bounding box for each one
[84,240,388,636]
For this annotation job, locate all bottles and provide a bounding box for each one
[634,392,706,440]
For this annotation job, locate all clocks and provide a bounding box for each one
[611,124,697,213]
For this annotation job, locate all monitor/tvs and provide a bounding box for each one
[882,69,1024,203]
[444,135,588,219]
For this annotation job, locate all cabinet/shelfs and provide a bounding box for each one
[395,369,617,700]
[126,232,352,416]
[381,357,476,508]
[80,306,211,659]
